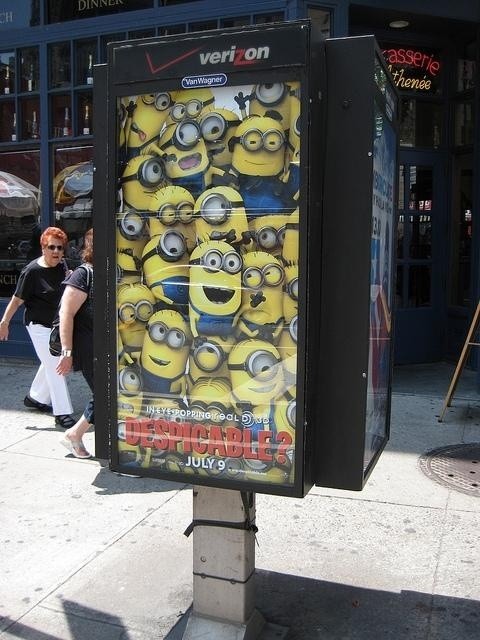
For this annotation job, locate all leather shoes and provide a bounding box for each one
[25,395,53,413]
[55,415,76,428]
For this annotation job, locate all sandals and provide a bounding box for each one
[60,434,91,459]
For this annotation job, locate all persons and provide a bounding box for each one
[57,227,94,459]
[0,227,76,429]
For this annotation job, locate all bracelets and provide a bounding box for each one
[60,349,73,358]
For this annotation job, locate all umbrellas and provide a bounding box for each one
[0,171,41,219]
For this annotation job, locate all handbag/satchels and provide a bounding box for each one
[49,264,94,358]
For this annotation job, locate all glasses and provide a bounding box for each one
[46,245,63,251]
